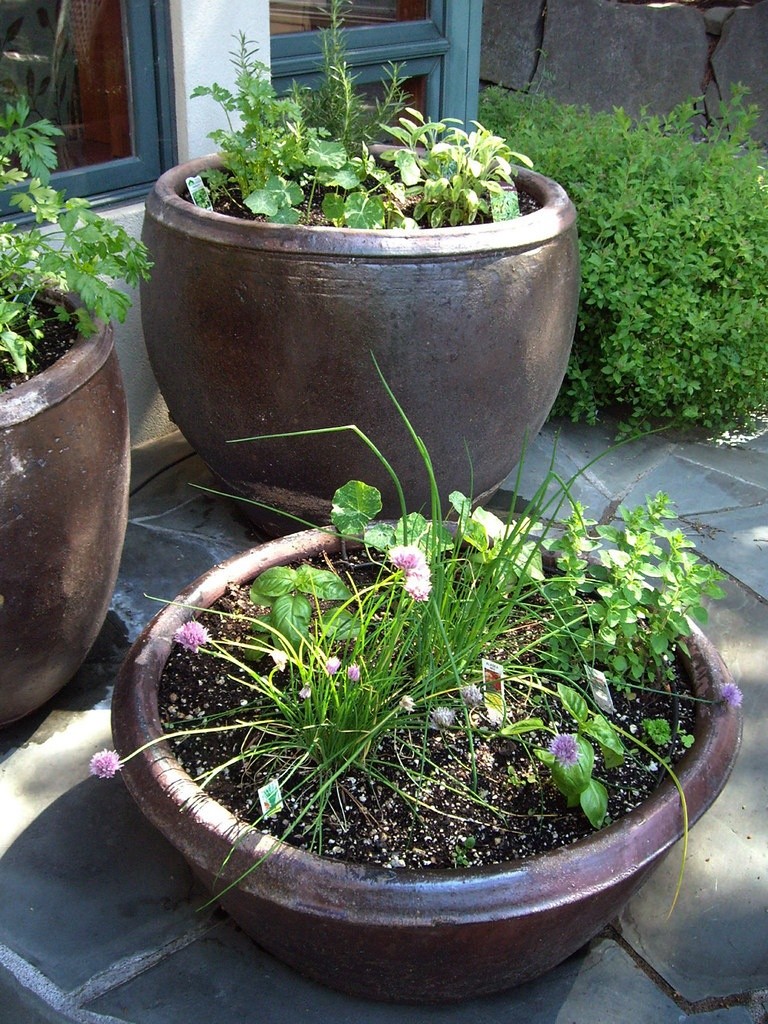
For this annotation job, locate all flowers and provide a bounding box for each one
[88,352,745,923]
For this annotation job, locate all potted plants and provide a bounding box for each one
[137,0,582,544]
[1,94,156,729]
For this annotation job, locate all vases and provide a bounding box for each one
[111,516,745,1009]
